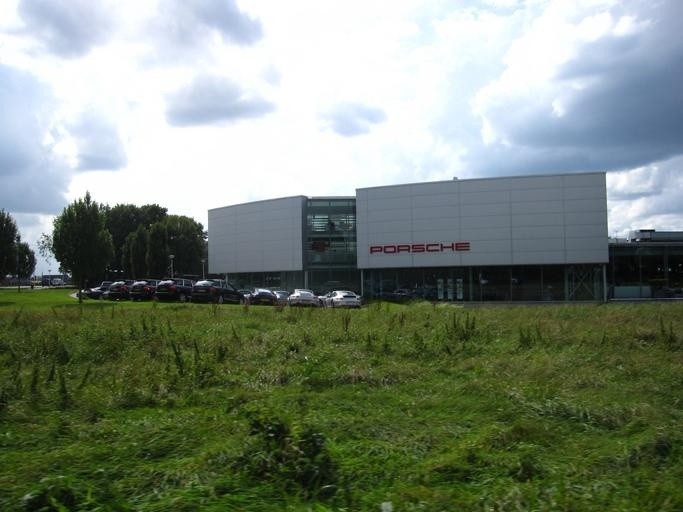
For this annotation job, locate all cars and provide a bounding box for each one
[52,278,66,286]
[392,288,412,296]
[86,277,328,307]
[325,289,363,308]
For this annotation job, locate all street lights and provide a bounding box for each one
[200,259,206,281]
[168,254,176,278]
[16,231,22,293]
[48,270,51,287]
